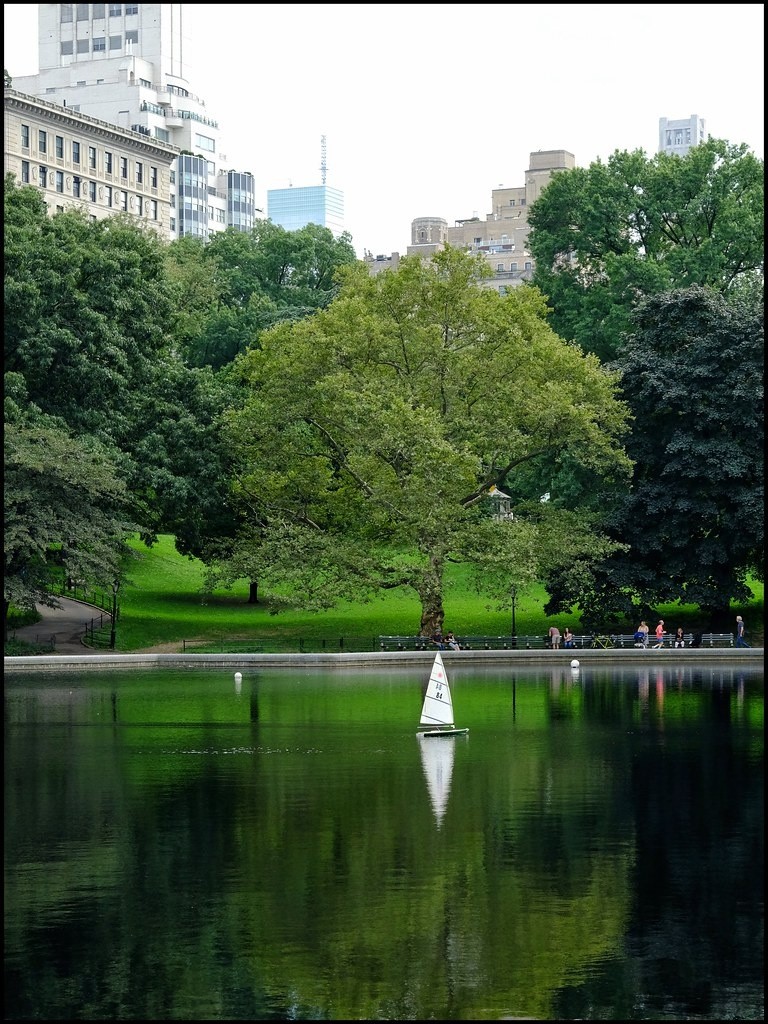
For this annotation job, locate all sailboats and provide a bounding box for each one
[416,650,470,737]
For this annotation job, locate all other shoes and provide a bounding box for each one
[652,646,655,648]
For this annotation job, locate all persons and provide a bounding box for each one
[563,627,573,648]
[652,620,666,649]
[736,616,751,648]
[549,626,560,649]
[674,628,685,648]
[433,628,444,650]
[444,631,460,651]
[638,621,649,648]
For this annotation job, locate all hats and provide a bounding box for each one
[659,620,663,623]
[435,629,441,631]
[449,631,453,633]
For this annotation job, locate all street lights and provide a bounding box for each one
[109,577,121,649]
[508,585,518,636]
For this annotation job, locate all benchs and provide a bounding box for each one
[378,633,734,648]
